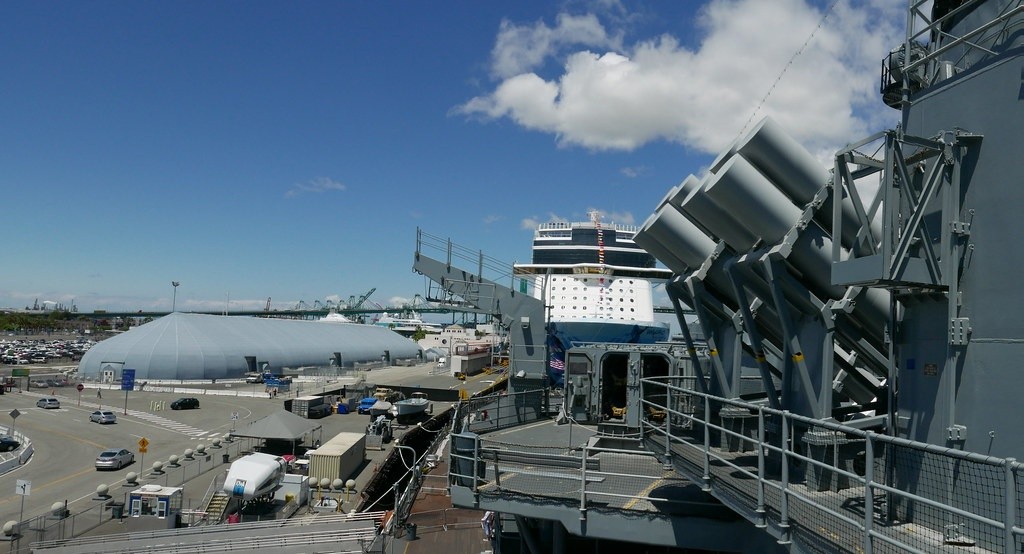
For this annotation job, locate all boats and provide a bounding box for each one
[368,399,389,416]
[392,397,431,415]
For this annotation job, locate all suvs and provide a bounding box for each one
[358,397,376,414]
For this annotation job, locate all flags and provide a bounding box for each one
[549,356,565,370]
[597,224,605,286]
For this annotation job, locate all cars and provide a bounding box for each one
[0,337,100,365]
[36,398,60,409]
[95,448,134,471]
[170,397,201,410]
[89,410,117,424]
[247,374,264,382]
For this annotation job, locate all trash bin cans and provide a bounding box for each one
[113,502,124,517]
[222,454,229,463]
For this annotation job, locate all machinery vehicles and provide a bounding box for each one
[362,415,393,451]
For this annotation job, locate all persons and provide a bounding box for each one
[97,388,101,399]
